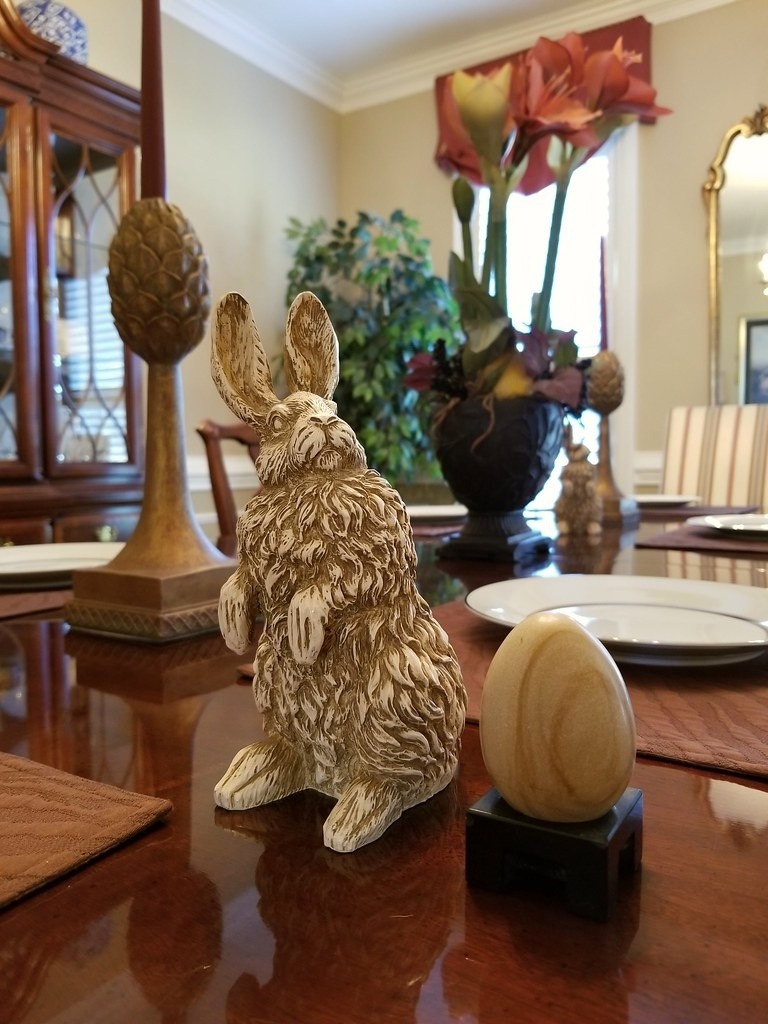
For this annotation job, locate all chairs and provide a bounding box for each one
[663,404,768,517]
[196,420,262,558]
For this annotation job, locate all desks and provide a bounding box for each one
[1,509,768,1024]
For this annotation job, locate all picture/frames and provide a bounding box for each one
[738,315,768,405]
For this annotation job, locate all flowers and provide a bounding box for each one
[403,30,674,456]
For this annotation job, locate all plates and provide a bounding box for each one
[0,542,125,587]
[685,514,767,532]
[406,505,468,520]
[635,493,698,505]
[464,574,768,666]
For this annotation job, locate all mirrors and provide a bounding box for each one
[702,104,768,405]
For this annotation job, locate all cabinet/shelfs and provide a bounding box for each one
[0,0,148,547]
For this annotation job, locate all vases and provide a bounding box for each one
[431,400,567,557]
